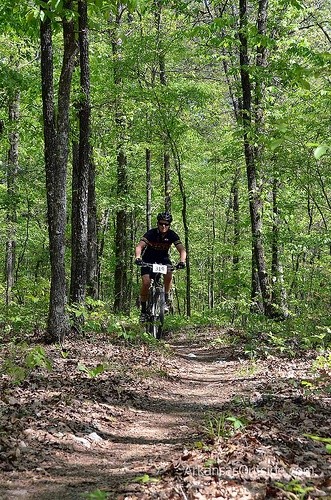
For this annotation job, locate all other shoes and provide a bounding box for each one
[139,311,145,322]
[166,293,173,302]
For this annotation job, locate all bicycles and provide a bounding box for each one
[133,260,186,340]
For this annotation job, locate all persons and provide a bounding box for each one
[135,213,186,322]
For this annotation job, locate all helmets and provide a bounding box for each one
[157,212,173,222]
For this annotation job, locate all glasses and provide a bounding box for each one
[159,222,170,226]
